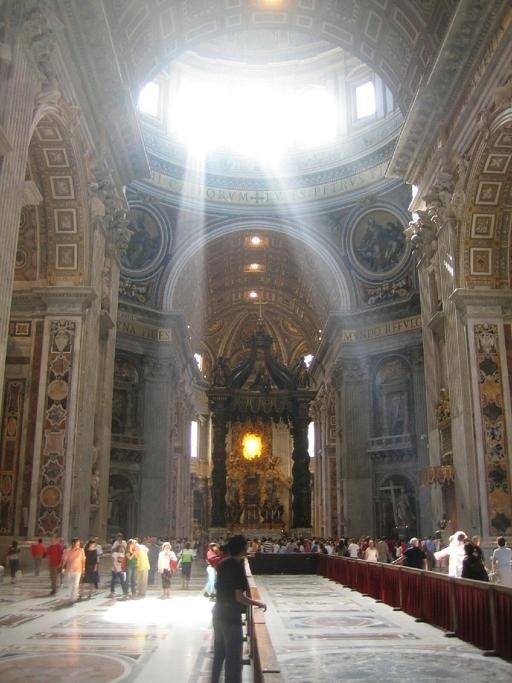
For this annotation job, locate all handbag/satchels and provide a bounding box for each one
[120,559,127,573]
[488,572,501,583]
[170,559,177,570]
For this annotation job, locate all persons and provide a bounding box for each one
[7,540,21,583]
[395,488,416,527]
[46,532,201,604]
[357,216,401,273]
[31,538,46,577]
[210,534,267,683]
[204,530,512,598]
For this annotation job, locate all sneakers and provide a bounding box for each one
[48,585,217,606]
